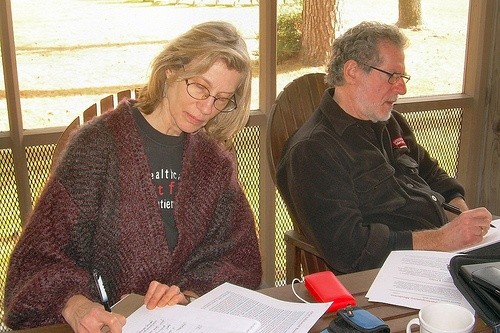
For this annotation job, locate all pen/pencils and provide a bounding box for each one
[93,269,111,312]
[442,202,496,228]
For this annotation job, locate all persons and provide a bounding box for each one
[4,21,263,333]
[274,20,492,276]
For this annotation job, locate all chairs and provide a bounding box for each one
[266,72,327,285]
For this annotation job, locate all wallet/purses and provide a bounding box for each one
[330,309,390,333]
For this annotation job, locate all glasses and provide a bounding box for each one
[180,63,238,113]
[362,63,410,85]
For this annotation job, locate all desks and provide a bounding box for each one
[3,268,493,333]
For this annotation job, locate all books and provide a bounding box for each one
[122,301,261,333]
[457,219,500,252]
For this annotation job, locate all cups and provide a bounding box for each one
[407,303,475,333]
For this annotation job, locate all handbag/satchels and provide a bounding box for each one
[450,255,499,330]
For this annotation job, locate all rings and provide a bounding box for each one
[479,226,483,235]
[179,290,180,294]
